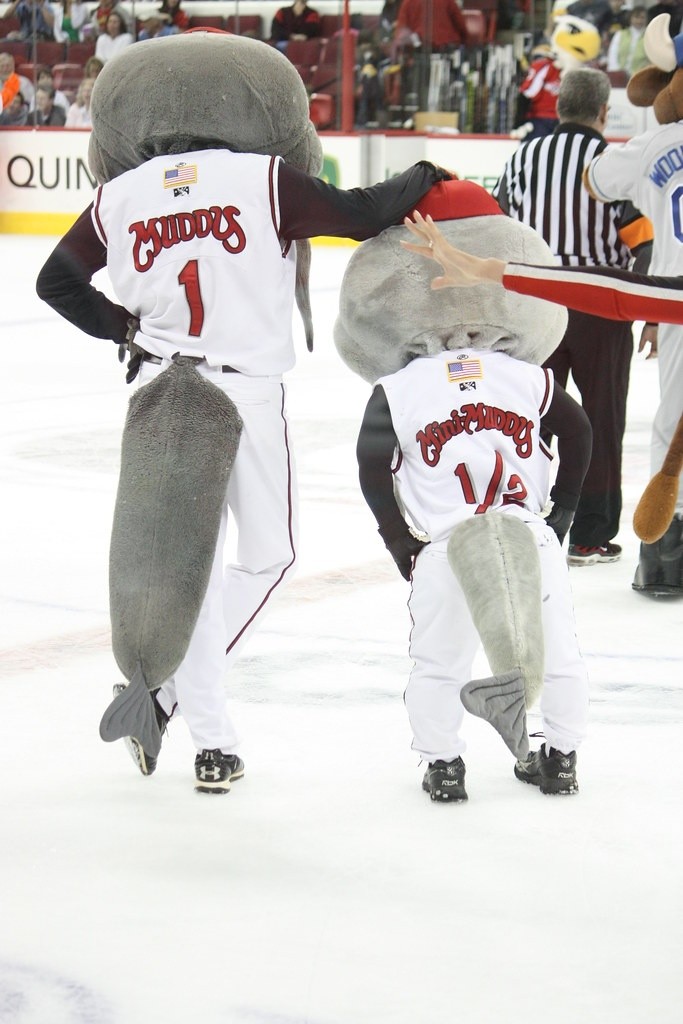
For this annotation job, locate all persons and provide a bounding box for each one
[399,210,683,326]
[491,69,657,563]
[0,0,683,138]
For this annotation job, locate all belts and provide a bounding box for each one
[142,351,240,373]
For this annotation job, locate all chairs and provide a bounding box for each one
[0,10,488,131]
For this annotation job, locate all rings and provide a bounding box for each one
[428,241,432,249]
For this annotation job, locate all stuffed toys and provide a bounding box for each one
[582,12,683,599]
[333,178,591,802]
[35,24,464,790]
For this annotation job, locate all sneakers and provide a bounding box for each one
[567,540,622,566]
[194,748,244,794]
[114,683,169,775]
[423,756,468,802]
[514,744,578,795]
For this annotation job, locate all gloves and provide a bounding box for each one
[385,530,430,581]
[117,315,144,384]
[544,502,575,547]
